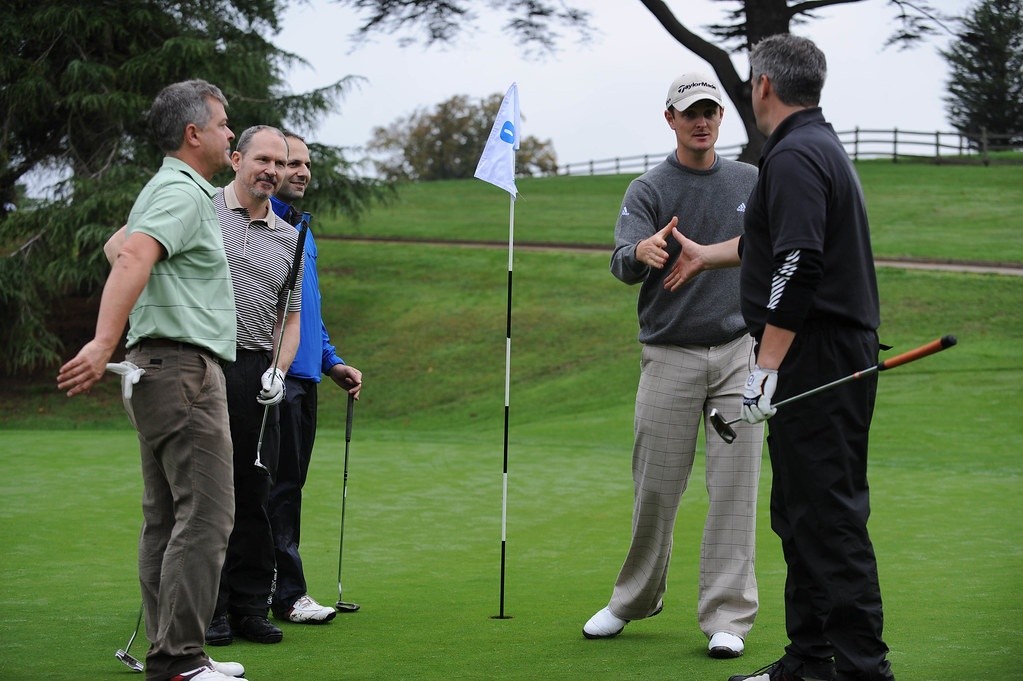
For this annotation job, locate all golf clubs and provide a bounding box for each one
[115,600,144,671]
[336,385,360,611]
[709,334,957,444]
[254,211,311,476]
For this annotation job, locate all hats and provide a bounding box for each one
[666,71,724,112]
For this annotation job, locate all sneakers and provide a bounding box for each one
[145,655,250,681]
[272,592,336,622]
[204,610,232,645]
[582,597,663,639]
[706,632,744,657]
[230,615,283,643]
[727,653,837,681]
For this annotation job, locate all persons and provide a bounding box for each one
[583,70,760,658]
[55,79,364,681]
[664,35,895,681]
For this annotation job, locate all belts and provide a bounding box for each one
[128,337,214,357]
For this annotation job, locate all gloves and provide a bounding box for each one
[256,366,287,406]
[106,360,146,400]
[739,365,779,425]
